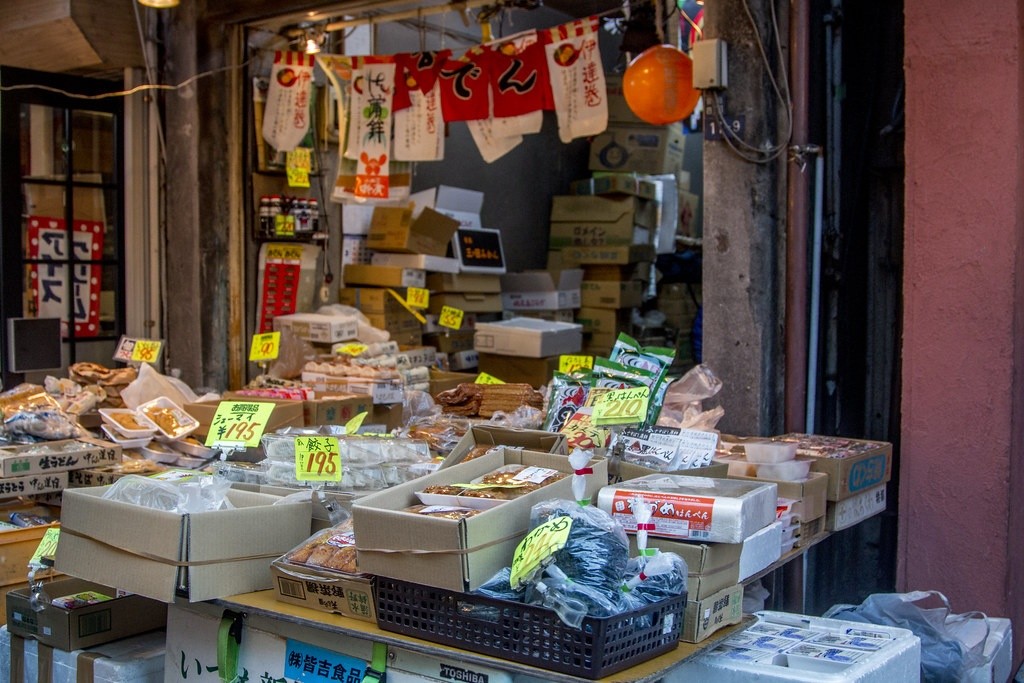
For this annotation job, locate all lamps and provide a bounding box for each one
[303,33,320,54]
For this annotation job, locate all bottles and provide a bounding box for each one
[259,194,319,234]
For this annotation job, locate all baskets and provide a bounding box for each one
[376,576,688,680]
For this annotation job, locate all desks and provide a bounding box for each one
[176,529,833,683]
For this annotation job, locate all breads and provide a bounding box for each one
[1,354,884,576]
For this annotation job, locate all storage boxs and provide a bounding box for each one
[0,73,1024,683]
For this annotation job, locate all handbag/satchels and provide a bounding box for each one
[824,589,990,683]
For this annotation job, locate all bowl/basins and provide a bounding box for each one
[777,498,802,555]
[711,439,818,482]
